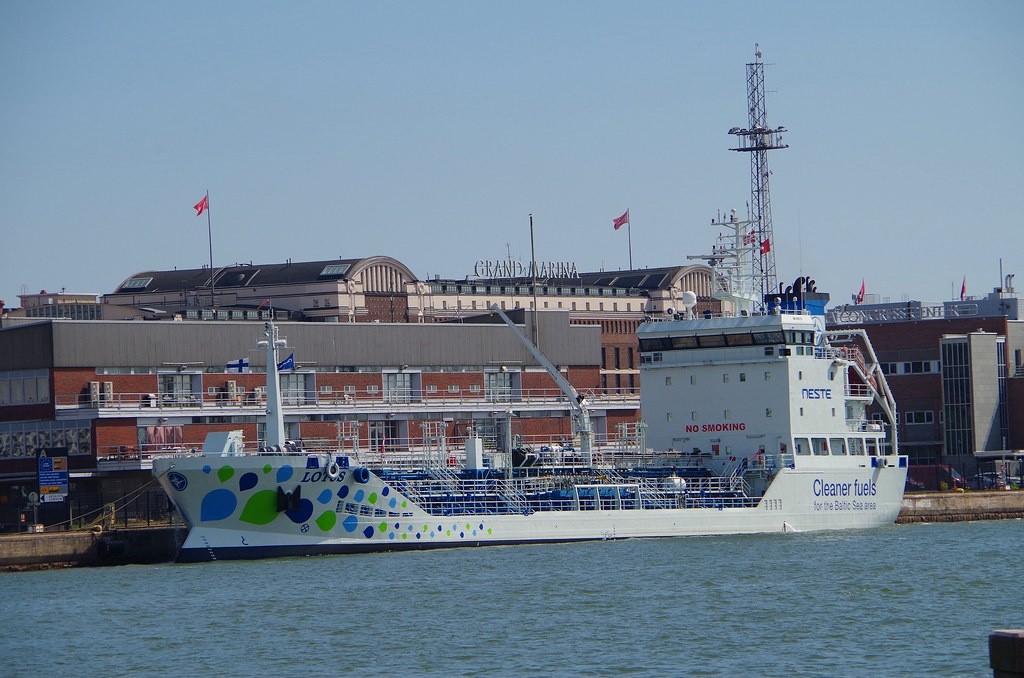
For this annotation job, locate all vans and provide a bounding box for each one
[905,465,968,492]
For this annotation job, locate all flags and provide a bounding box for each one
[961,280,966,301]
[743,230,770,254]
[278,353,294,372]
[226,357,249,373]
[858,280,865,304]
[194,195,208,216]
[613,211,628,230]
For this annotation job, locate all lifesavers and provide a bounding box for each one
[358,467,370,484]
[880,457,888,469]
[327,461,340,478]
[869,376,877,388]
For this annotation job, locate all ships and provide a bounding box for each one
[152,201,908,566]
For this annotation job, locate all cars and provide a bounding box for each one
[967,470,1022,491]
